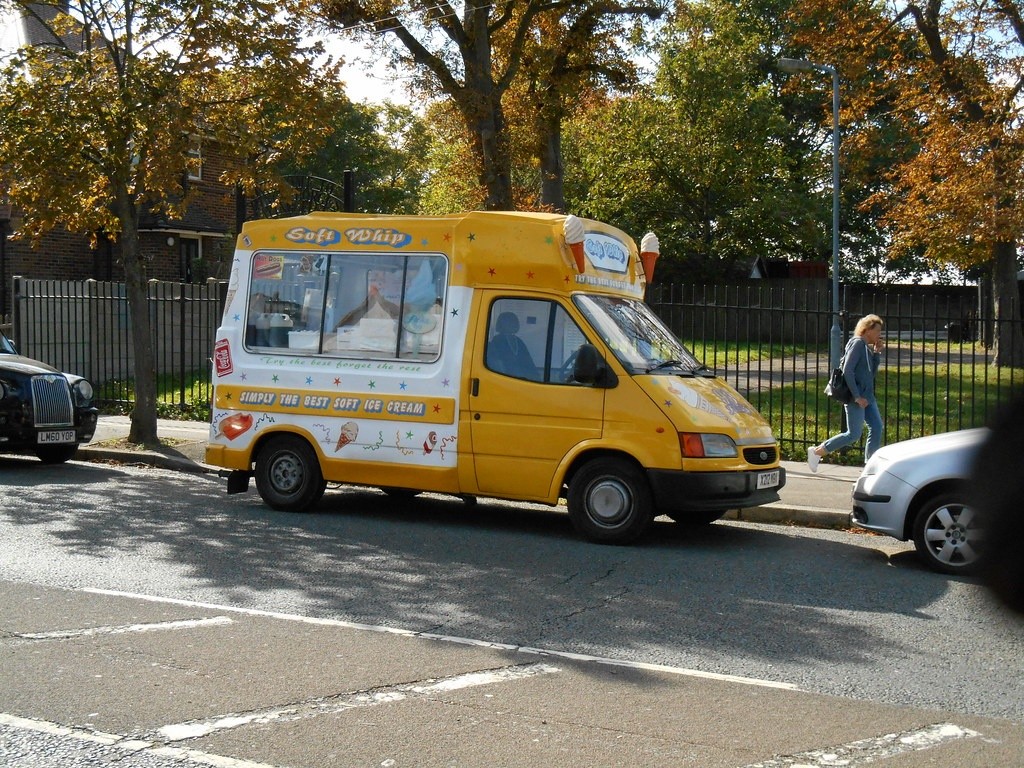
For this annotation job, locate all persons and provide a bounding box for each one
[808,315,884,472]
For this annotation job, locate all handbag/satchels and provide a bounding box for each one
[823,369,859,404]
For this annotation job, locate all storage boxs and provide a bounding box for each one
[288,331,320,350]
[336,325,359,350]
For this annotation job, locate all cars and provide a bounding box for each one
[849,427,1000,576]
[0,330,99,462]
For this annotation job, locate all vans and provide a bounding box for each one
[945,304,980,341]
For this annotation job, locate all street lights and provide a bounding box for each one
[776,58,841,372]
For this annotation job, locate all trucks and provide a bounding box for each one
[201,211,786,545]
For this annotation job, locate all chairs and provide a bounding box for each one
[491,312,539,382]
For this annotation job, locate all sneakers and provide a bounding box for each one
[807,447,821,472]
